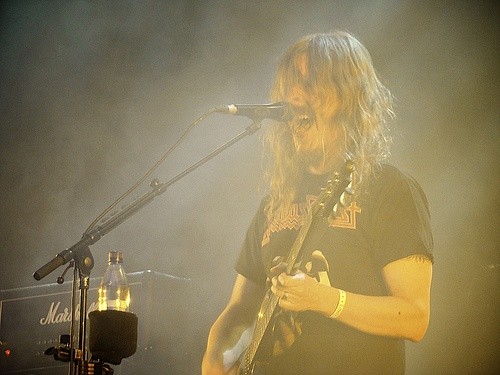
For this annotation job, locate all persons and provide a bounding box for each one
[200,32,437,374]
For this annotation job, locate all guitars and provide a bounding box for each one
[225,159,355,374]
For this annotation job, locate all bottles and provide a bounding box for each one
[98,250,130,313]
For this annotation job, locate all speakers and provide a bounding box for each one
[0,270,194,375]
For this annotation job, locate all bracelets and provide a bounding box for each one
[326,288,346,319]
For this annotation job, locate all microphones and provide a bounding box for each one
[214,101,294,121]
